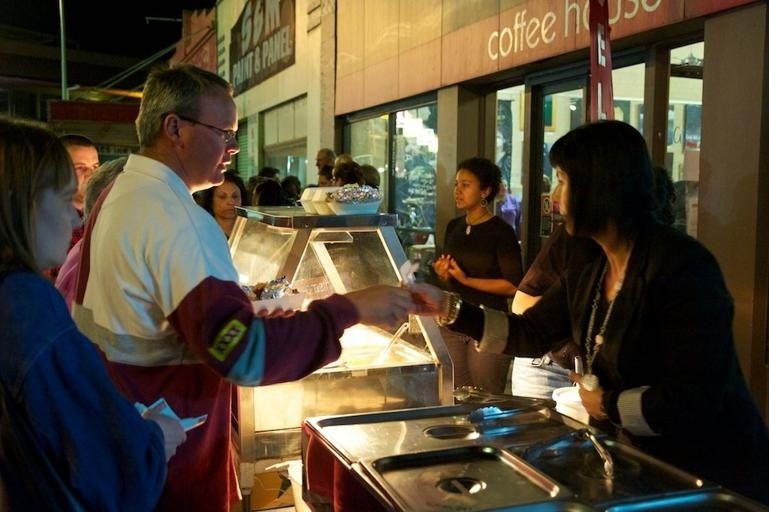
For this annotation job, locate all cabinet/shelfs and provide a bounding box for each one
[221,205,454,462]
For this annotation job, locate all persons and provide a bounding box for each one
[71,65,418,512]
[42,133,100,284]
[55,158,128,314]
[0,116,187,512]
[432,157,523,394]
[511,165,676,371]
[202,148,380,240]
[401,121,769,507]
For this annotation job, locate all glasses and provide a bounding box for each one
[160,111,237,144]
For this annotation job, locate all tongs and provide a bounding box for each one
[449,384,557,424]
